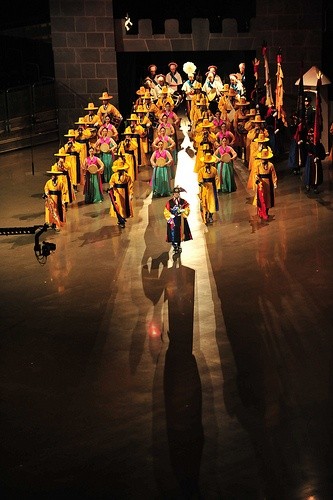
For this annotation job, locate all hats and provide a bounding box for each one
[198,119,213,127]
[159,86,169,93]
[172,188,182,193]
[219,84,229,91]
[136,87,154,99]
[225,89,238,96]
[46,103,98,173]
[194,83,203,89]
[257,149,273,159]
[229,74,238,81]
[193,93,209,106]
[98,92,112,99]
[143,63,178,88]
[113,105,148,170]
[250,115,266,122]
[235,98,250,105]
[254,133,270,142]
[199,152,216,163]
[205,66,217,78]
[246,109,256,116]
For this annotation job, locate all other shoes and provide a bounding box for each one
[209,212,213,222]
[291,172,302,176]
[173,246,182,253]
[314,190,319,194]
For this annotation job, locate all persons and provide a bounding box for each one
[94,117,118,183]
[41,166,67,232]
[82,146,104,205]
[250,88,333,193]
[252,149,278,221]
[96,91,122,129]
[197,153,220,225]
[107,160,134,228]
[150,141,173,198]
[213,136,237,193]
[164,186,193,254]
[118,62,273,190]
[51,102,103,212]
